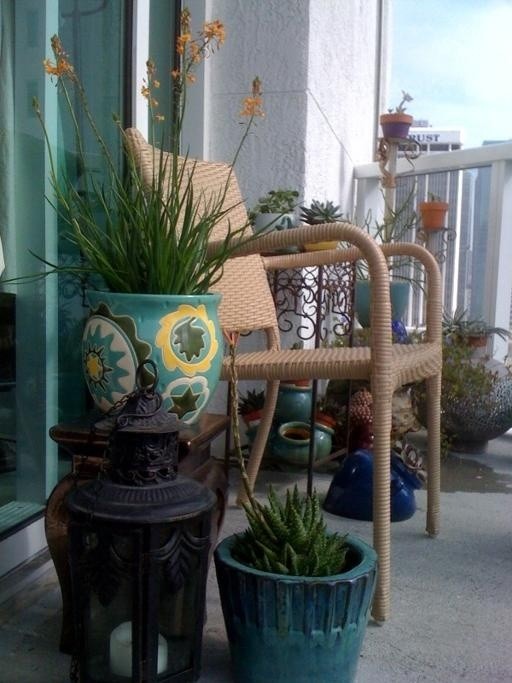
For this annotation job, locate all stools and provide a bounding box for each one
[42,411,230,657]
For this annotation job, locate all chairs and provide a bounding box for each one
[120,124,443,624]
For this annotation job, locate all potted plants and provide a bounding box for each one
[211,480,380,682]
[378,88,414,135]
[236,185,512,455]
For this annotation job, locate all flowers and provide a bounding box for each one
[0,6,305,294]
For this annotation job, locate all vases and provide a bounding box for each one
[268,421,317,471]
[81,291,227,426]
